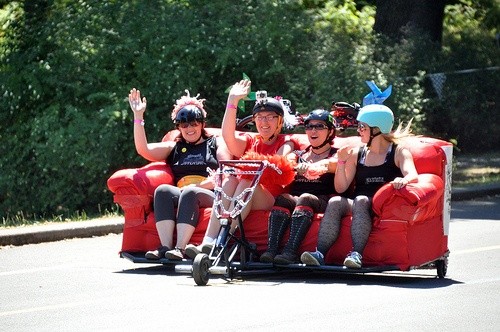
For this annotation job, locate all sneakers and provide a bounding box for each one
[343,251,362,269]
[300,250,325,266]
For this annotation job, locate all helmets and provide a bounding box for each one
[356,103,395,135]
[252,97,284,117]
[303,108,337,132]
[175,104,204,123]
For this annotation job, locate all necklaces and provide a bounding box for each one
[311,147,330,158]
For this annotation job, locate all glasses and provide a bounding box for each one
[304,122,329,131]
[180,121,201,128]
[253,114,280,121]
[357,123,370,132]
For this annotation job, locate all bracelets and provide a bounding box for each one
[337,160,346,163]
[135,119,144,124]
[227,105,237,110]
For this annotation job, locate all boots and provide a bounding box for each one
[260,210,290,264]
[272,209,314,265]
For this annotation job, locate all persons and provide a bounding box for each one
[260,109,348,264]
[301,104,419,268]
[129,88,233,260]
[184,79,302,259]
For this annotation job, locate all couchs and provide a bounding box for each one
[107,128,453,278]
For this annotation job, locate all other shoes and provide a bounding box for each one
[202,244,221,257]
[165,247,185,261]
[185,244,201,260]
[145,246,170,259]
